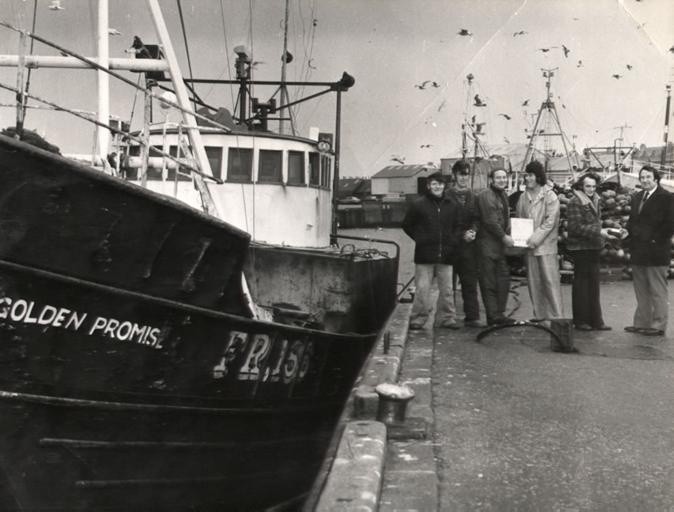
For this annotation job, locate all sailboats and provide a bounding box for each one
[0,0,401,512]
[462,67,674,207]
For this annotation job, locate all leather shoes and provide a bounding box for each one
[409,316,664,335]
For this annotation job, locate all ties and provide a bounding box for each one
[643,192,649,201]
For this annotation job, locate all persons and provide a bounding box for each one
[623,165,674,337]
[402,171,467,330]
[474,167,516,325]
[515,160,563,329]
[443,159,487,328]
[565,172,613,331]
